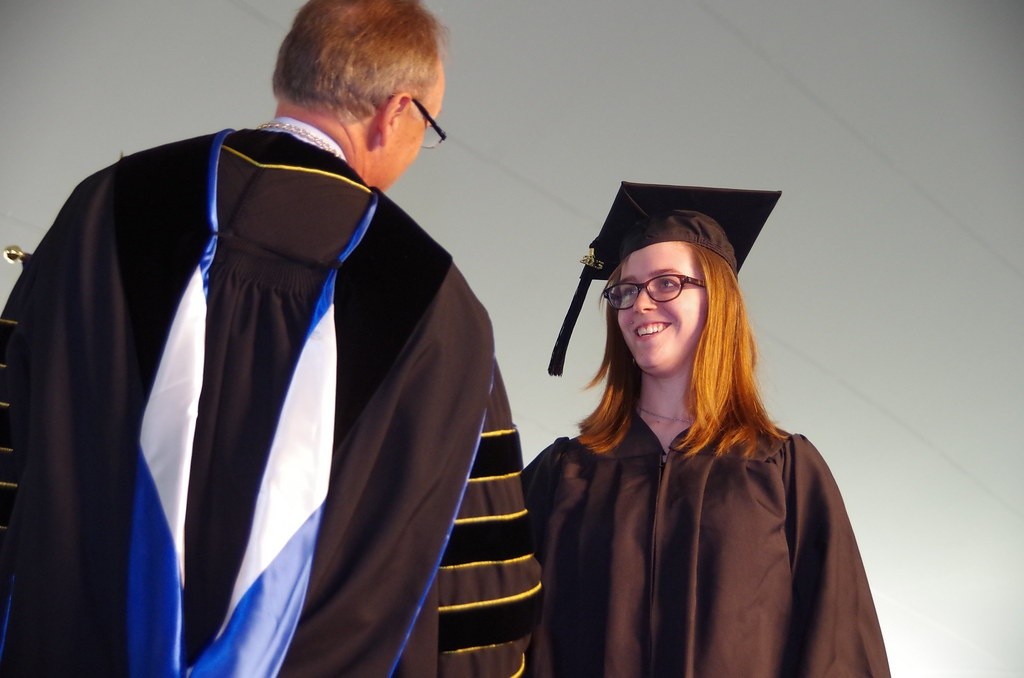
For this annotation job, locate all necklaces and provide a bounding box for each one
[255,122,346,163]
[635,405,692,426]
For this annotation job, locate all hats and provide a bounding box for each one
[546,182,783,376]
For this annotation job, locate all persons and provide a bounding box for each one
[0,2,547,677]
[522,182,890,678]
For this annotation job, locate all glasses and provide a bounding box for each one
[602,274,706,309]
[388,93,446,149]
